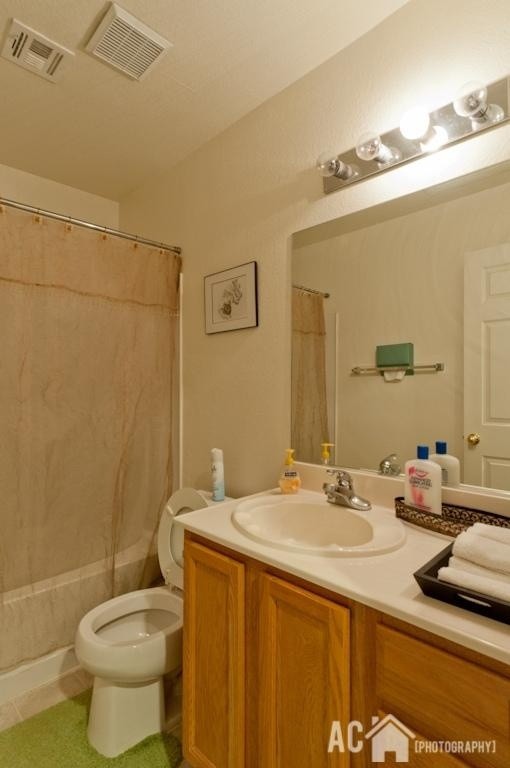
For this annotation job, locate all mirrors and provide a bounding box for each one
[290,156,510,501]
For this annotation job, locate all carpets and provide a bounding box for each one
[1,688,183,767]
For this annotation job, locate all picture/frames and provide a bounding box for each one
[204,260,258,336]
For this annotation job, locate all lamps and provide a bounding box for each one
[399,107,445,150]
[450,81,501,127]
[313,153,354,182]
[377,149,401,170]
[470,104,503,129]
[418,126,446,151]
[354,131,397,165]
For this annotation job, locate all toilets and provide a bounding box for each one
[75,488,238,759]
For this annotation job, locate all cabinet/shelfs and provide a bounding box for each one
[178,532,367,768]
[368,603,510,768]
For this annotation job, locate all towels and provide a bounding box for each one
[436,521,510,606]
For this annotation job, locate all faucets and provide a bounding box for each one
[322,468,371,510]
[380,454,401,477]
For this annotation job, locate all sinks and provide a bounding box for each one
[232,492,407,556]
